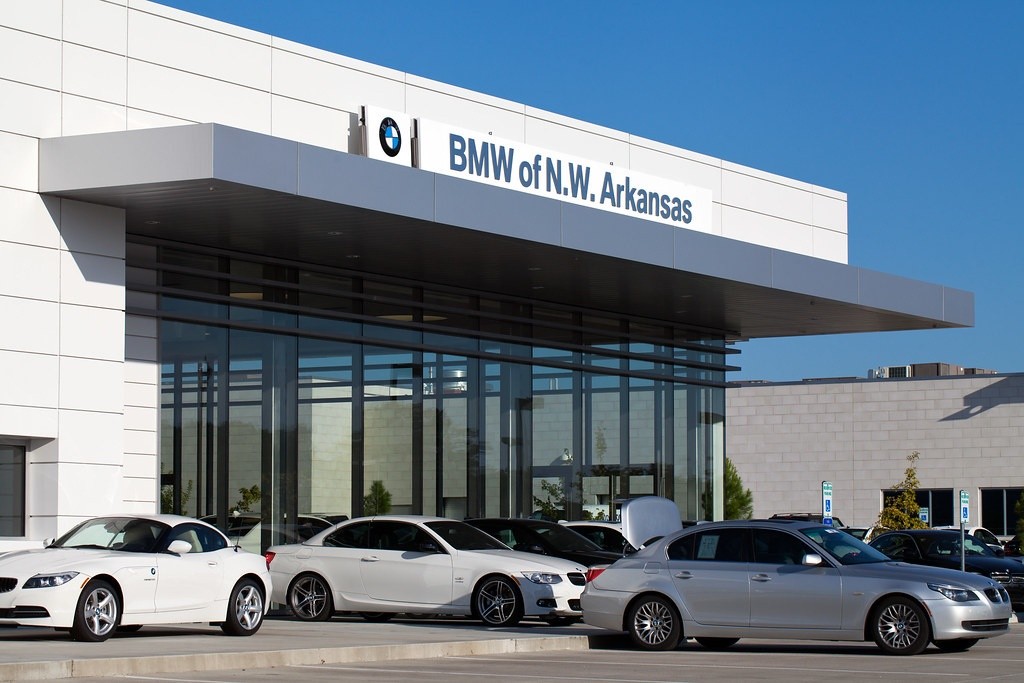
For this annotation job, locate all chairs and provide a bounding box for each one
[174,529,202,551]
[123,524,155,552]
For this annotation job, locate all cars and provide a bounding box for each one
[266,514,589,627]
[0,512,274,643]
[453,516,647,581]
[193,514,335,555]
[581,495,1011,652]
[768,514,1024,617]
